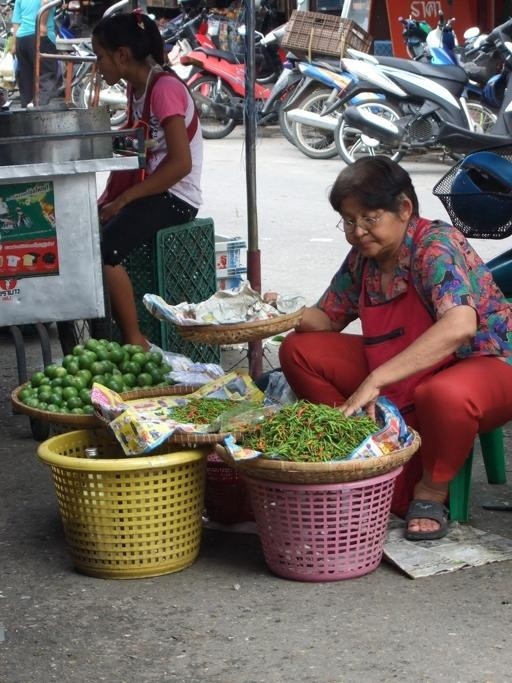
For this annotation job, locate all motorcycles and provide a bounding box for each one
[0,0,303,137]
[263,15,433,148]
[334,20,511,164]
[436,150,511,296]
[294,10,498,162]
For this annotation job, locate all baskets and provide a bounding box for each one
[38,422,405,583]
[282,10,372,58]
[432,142,512,239]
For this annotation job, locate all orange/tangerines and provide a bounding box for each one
[19,339,167,415]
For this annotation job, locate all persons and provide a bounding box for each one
[264,153,512,538]
[12,0,56,111]
[90,9,204,354]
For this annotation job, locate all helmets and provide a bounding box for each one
[453,153,512,231]
[484,74,504,108]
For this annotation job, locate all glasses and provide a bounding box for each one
[336,208,387,232]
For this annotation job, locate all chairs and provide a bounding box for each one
[449,296,512,524]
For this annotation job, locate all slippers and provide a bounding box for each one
[408,499,449,541]
